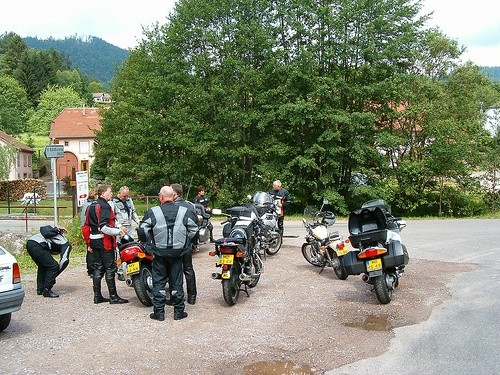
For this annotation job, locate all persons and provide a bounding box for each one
[194,184,216,243]
[136,186,200,321]
[170,183,197,305]
[85,184,129,304]
[111,185,140,281]
[269,180,290,244]
[26,232,72,297]
[80,188,105,279]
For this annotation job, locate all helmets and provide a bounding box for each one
[321,211,335,226]
[229,228,247,239]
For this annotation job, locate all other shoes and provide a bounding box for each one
[174,312,188,320]
[37,289,42,295]
[118,274,125,280]
[210,237,216,243]
[42,288,59,297]
[188,296,196,304]
[94,295,109,304]
[150,313,164,321]
[110,294,128,304]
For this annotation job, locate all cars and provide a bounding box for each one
[0,245,26,332]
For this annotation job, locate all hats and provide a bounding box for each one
[171,183,183,197]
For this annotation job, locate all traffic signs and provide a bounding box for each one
[44,145,64,158]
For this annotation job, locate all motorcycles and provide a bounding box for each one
[114,227,156,308]
[334,198,409,304]
[301,197,350,280]
[191,205,215,252]
[210,190,283,306]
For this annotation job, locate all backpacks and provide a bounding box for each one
[40,225,60,239]
